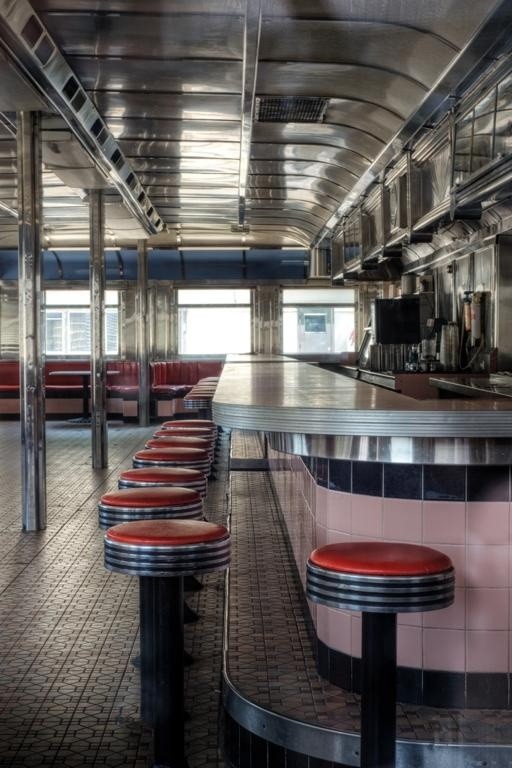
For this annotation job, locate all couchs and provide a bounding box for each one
[0,359,221,420]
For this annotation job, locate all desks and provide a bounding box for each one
[48,370,121,426]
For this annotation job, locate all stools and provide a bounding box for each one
[145,437,214,593]
[154,427,217,483]
[116,465,205,669]
[102,519,233,766]
[134,446,211,621]
[302,537,454,766]
[161,419,219,473]
[102,485,205,725]
